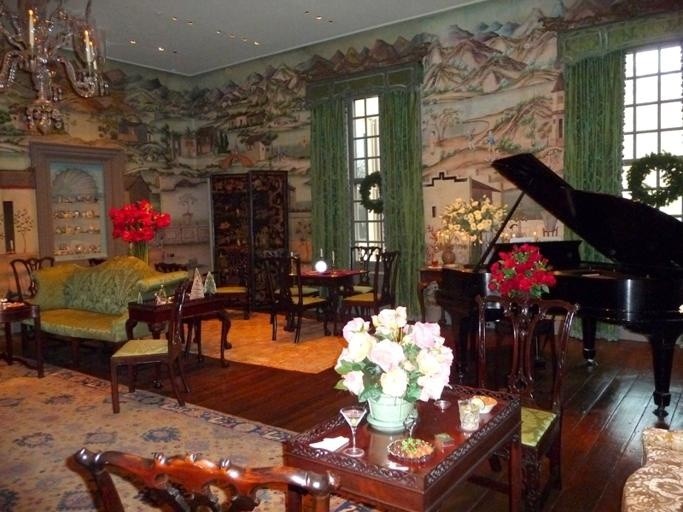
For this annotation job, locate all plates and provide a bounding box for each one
[387,438,437,463]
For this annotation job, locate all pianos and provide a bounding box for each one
[434,153,683,415]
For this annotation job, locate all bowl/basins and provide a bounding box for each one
[470,396,498,414]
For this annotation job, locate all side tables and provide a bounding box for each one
[125,295,235,367]
[0,299,46,379]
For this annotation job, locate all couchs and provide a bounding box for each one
[21,256,189,376]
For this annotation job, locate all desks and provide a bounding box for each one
[417,264,441,328]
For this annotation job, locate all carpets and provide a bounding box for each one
[178,305,348,375]
[0,357,505,512]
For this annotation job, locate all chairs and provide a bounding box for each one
[340,246,381,297]
[342,251,401,321]
[473,298,576,494]
[67,430,335,512]
[199,271,252,320]
[263,256,332,343]
[10,257,55,302]
[110,278,191,413]
[263,249,320,325]
[155,262,192,275]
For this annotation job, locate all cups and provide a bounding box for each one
[340,406,367,458]
[459,400,479,431]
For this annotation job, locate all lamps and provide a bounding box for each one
[0,1,110,137]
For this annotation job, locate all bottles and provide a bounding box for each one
[157,284,166,304]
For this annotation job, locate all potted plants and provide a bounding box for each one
[177,193,198,223]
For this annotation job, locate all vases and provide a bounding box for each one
[366,398,417,433]
[468,241,482,266]
[128,242,149,268]
[441,244,456,265]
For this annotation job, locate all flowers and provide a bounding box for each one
[333,308,453,402]
[108,199,172,243]
[437,227,459,245]
[440,194,518,243]
[488,245,557,301]
